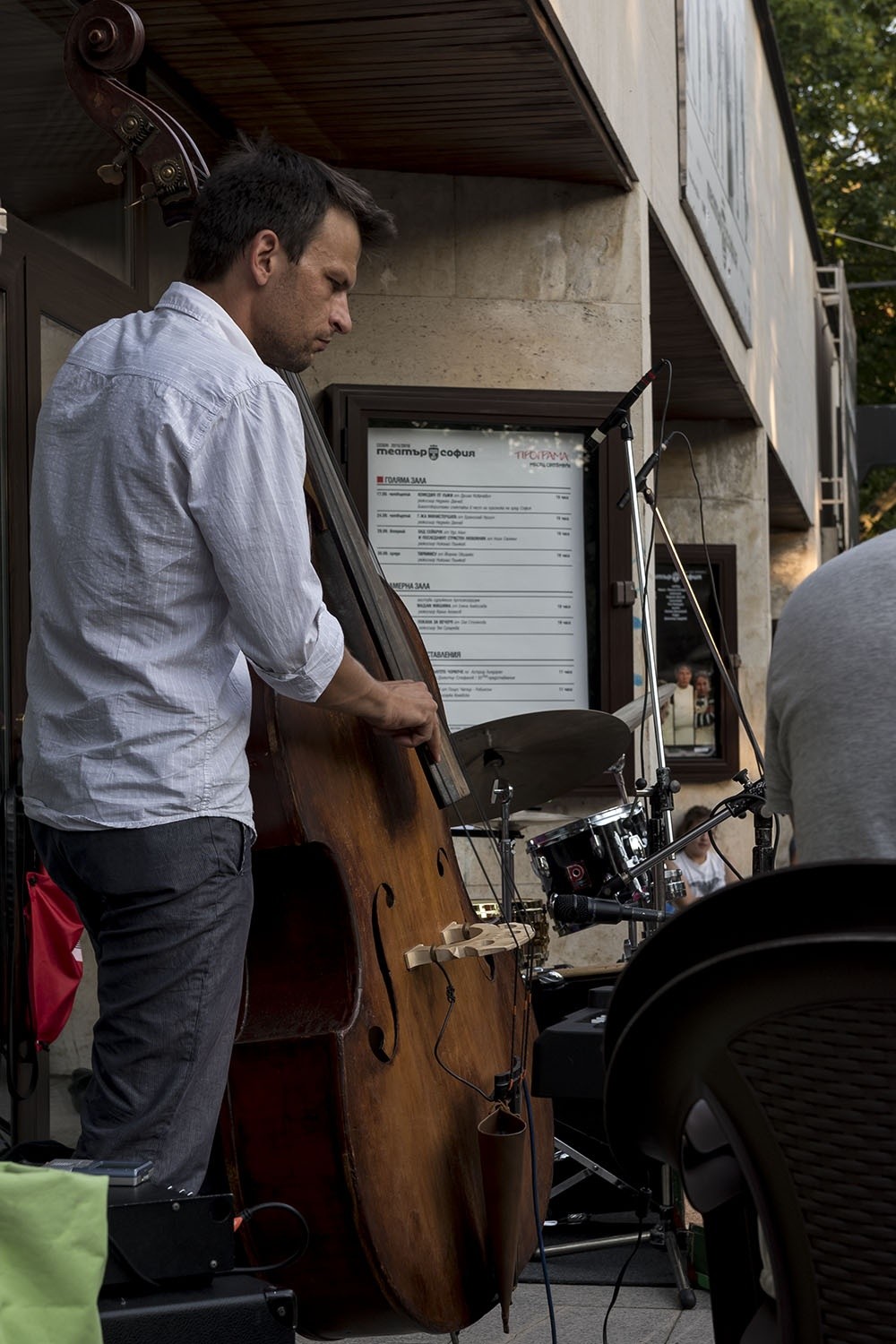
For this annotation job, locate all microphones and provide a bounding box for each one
[554,895,676,923]
[585,361,665,452]
[618,442,669,510]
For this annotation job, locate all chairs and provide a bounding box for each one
[599,859,896,1344]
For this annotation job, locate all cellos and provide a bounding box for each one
[66,0,554,1344]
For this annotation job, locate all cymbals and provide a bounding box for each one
[447,709,630,828]
[470,810,580,830]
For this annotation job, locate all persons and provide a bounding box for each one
[658,663,716,746]
[757,525,895,867]
[659,804,727,913]
[20,141,445,1200]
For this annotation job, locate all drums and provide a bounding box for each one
[524,799,687,938]
[470,898,549,970]
